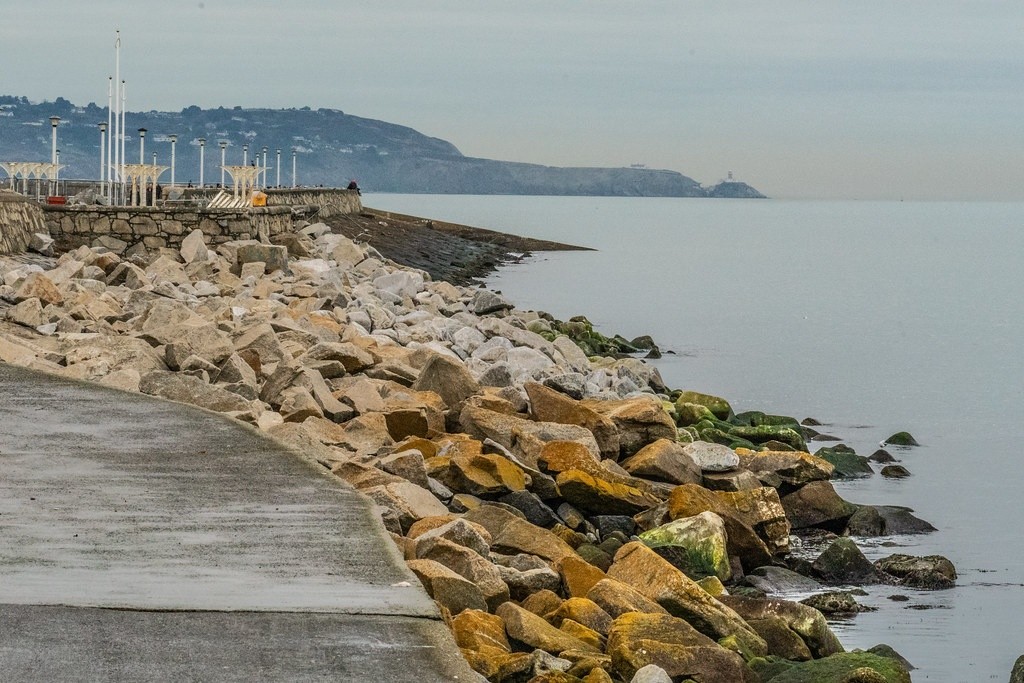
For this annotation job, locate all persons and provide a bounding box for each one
[347,180,362,196]
[130,183,162,206]
